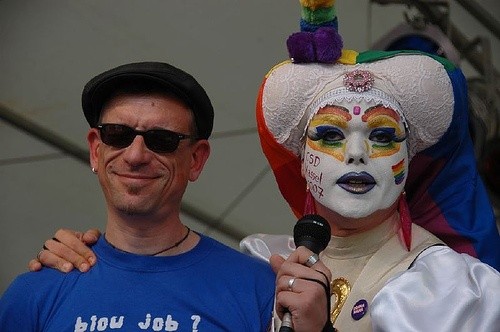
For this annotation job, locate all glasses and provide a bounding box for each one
[96,123,189,153]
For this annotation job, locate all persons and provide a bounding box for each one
[0,62,277,332]
[28,0,500,332]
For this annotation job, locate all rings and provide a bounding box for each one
[287,276,295,292]
[303,254,320,268]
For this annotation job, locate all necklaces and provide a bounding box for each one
[104,226,191,256]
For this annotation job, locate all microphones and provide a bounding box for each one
[279,213,332,332]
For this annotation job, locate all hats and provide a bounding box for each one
[81,62,214,138]
